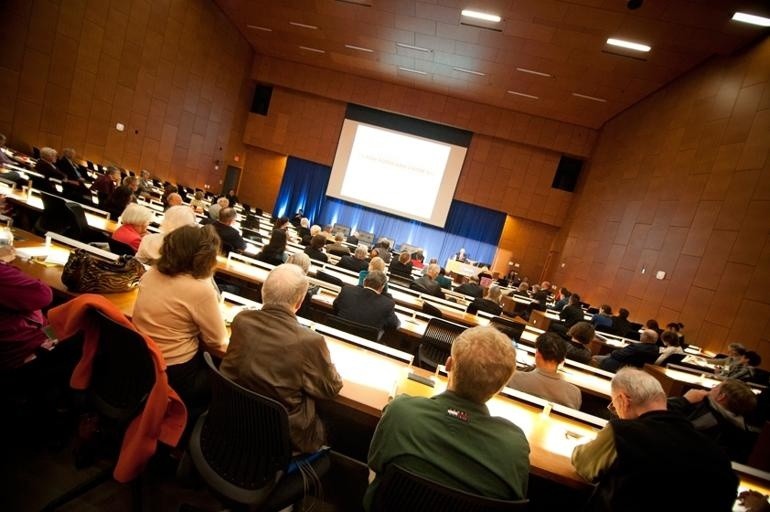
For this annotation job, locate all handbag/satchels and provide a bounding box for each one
[61,247,146,293]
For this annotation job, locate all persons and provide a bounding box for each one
[0,146,769,512]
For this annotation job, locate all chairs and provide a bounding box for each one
[0,138,770,512]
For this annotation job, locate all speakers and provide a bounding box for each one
[251,85,273,116]
[552,156,583,192]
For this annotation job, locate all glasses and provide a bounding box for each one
[607,392,632,416]
